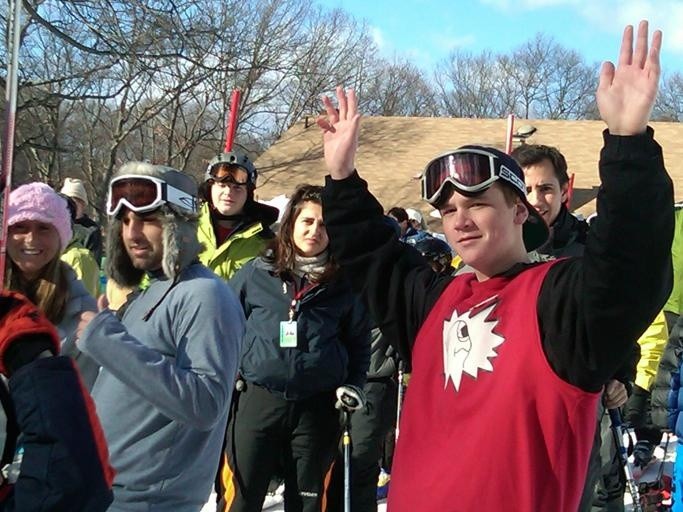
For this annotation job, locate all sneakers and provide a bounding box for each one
[589,482,624,512]
[633,428,661,466]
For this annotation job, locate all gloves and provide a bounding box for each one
[334,384,368,411]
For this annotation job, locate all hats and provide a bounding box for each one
[406,206,428,230]
[453,144,551,255]
[105,160,196,288]
[60,175,88,204]
[4,179,72,255]
[205,151,259,188]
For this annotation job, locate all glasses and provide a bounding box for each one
[106,174,200,216]
[206,163,253,188]
[417,149,527,203]
